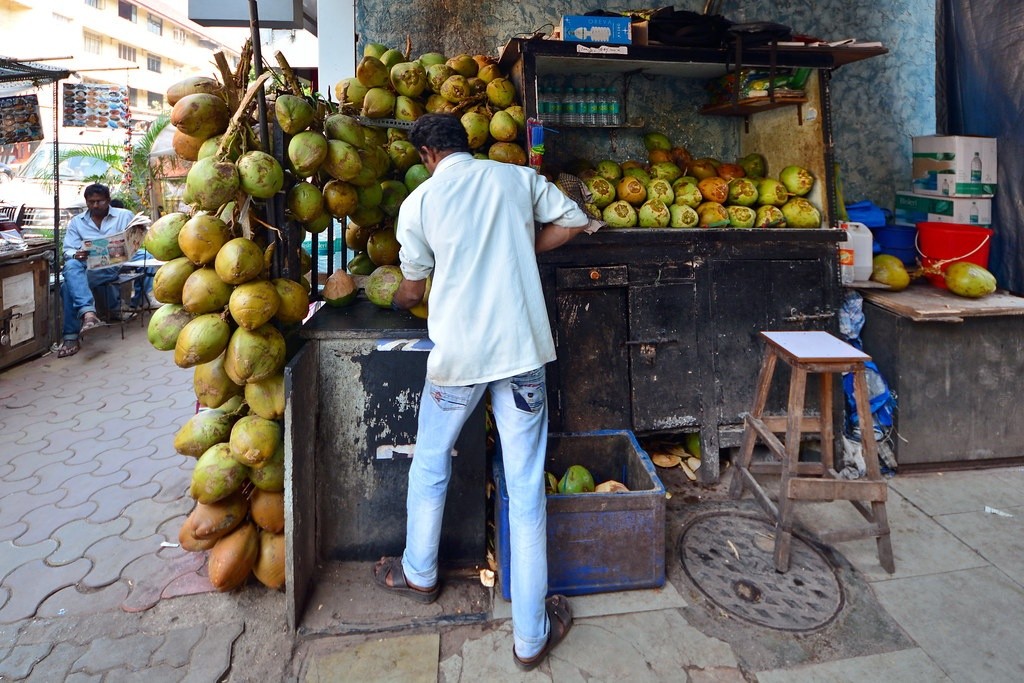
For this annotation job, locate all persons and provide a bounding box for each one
[57,183,136,356]
[97,197,163,322]
[368,110,587,671]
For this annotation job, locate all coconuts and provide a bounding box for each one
[564,132,822,229]
[871,254,910,291]
[945,262,997,297]
[142,40,531,592]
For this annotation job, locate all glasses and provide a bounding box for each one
[87,199,107,206]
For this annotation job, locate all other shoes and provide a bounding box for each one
[144,299,164,309]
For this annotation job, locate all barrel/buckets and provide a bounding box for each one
[915,222,994,290]
[874,226,919,266]
[838,220,873,281]
[844,200,885,255]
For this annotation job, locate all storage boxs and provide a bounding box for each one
[912,135,998,200]
[895,191,992,227]
[491,429,666,602]
[559,15,632,45]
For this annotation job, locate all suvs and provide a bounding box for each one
[0,129,146,244]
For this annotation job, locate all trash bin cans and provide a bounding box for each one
[489,431,668,602]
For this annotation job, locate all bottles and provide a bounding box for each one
[942,179,949,198]
[970,201,978,224]
[839,224,855,285]
[538,86,626,125]
[971,152,982,194]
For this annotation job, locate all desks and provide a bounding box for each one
[0,242,58,374]
[124,259,170,318]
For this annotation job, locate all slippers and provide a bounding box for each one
[57,340,80,357]
[374,556,444,604]
[79,317,107,335]
[513,594,572,672]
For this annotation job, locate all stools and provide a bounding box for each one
[729,331,896,575]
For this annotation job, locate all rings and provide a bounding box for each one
[79,256,83,258]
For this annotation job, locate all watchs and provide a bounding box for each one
[391,301,399,311]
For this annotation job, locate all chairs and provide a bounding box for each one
[81,244,146,341]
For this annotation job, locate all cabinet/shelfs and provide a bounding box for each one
[500,36,886,487]
[282,286,488,639]
